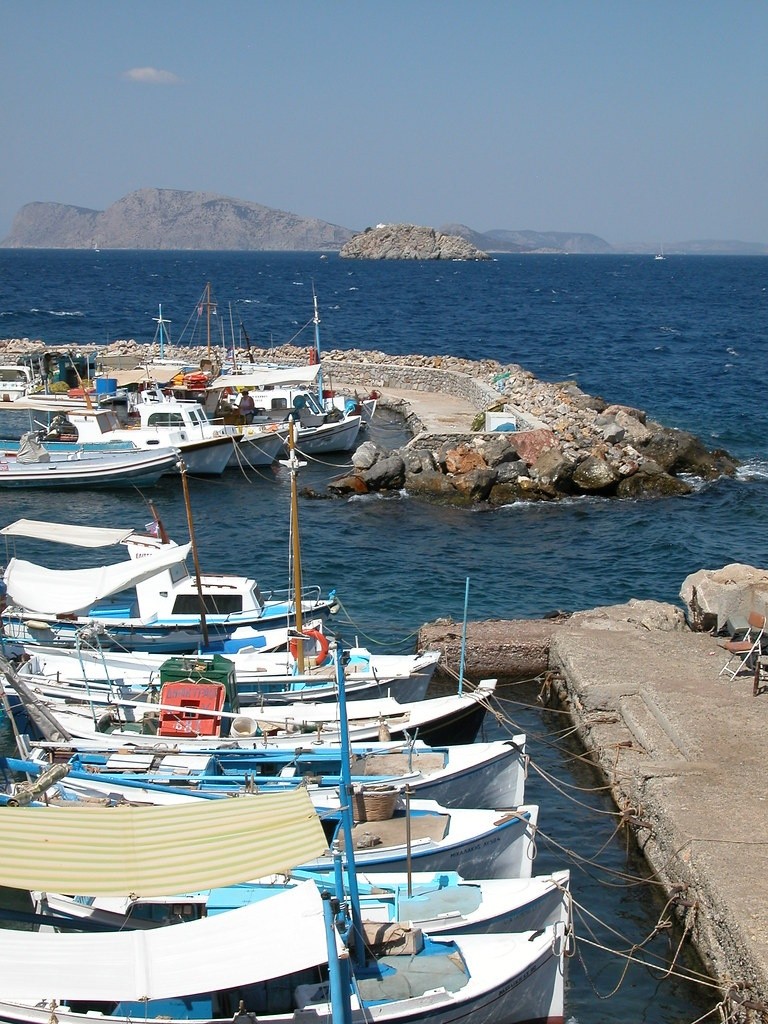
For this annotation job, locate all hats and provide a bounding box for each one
[240,391,249,394]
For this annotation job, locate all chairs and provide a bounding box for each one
[719,613,766,682]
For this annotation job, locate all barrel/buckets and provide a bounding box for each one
[230,716,257,738]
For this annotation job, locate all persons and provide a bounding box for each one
[238,391,254,425]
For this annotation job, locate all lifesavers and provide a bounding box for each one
[182,382,209,389]
[289,628,328,667]
[183,374,208,382]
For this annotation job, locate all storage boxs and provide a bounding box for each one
[486,412,516,433]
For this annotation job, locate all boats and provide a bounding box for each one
[0,279,579,1022]
